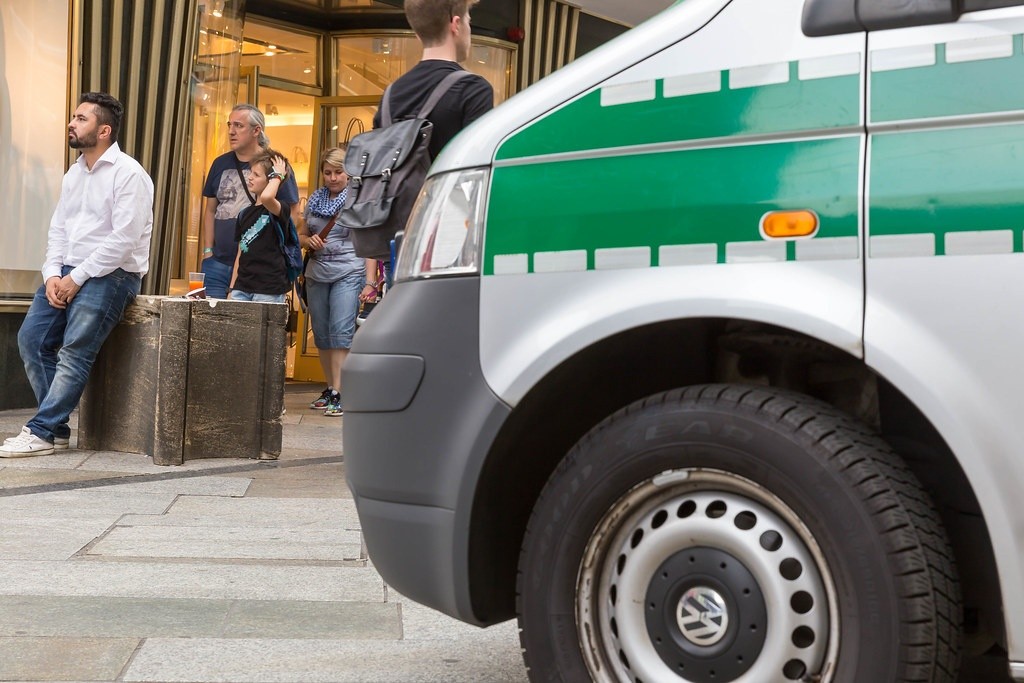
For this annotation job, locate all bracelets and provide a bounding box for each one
[202,248,212,255]
[225,287,233,294]
[267,173,285,182]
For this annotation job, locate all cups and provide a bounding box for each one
[188,272,206,292]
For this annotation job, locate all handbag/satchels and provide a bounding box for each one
[294,273,309,314]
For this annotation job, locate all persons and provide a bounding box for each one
[201,104,299,300]
[227,148,294,303]
[298,147,386,417]
[0,92,154,458]
[373,0,493,294]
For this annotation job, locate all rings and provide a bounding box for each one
[312,242,315,244]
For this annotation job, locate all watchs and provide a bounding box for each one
[365,279,378,288]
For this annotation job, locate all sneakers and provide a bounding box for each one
[0,425,55,458]
[309,385,333,410]
[324,388,343,416]
[53,436,69,449]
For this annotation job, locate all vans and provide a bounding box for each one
[336,0,1024,683]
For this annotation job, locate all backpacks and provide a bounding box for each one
[269,210,304,282]
[334,70,477,259]
